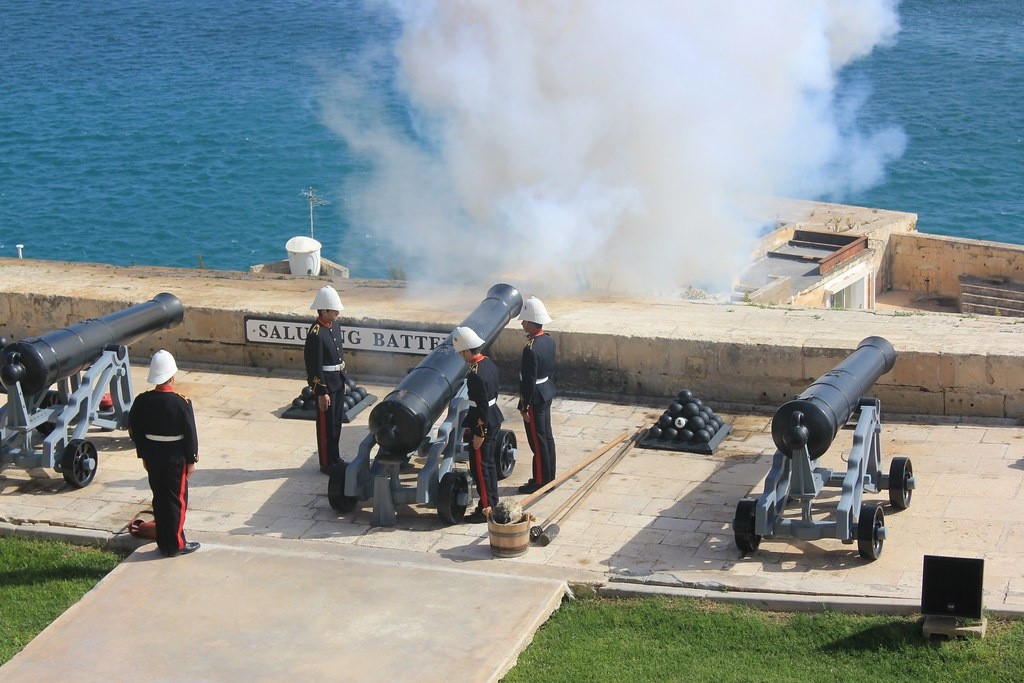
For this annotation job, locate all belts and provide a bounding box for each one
[520,372,548,384]
[146,434,184,441]
[469,398,496,407]
[323,361,345,371]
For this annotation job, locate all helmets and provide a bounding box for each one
[517,295,552,324]
[147,349,178,385]
[309,286,343,311]
[453,326,485,353]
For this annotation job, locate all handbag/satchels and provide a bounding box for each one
[128,510,156,539]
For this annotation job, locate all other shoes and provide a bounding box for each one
[320,459,348,476]
[519,479,553,494]
[161,541,201,558]
[463,510,488,524]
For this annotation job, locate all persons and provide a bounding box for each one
[304,286,346,475]
[453,327,504,523]
[128,350,200,557]
[517,296,556,494]
